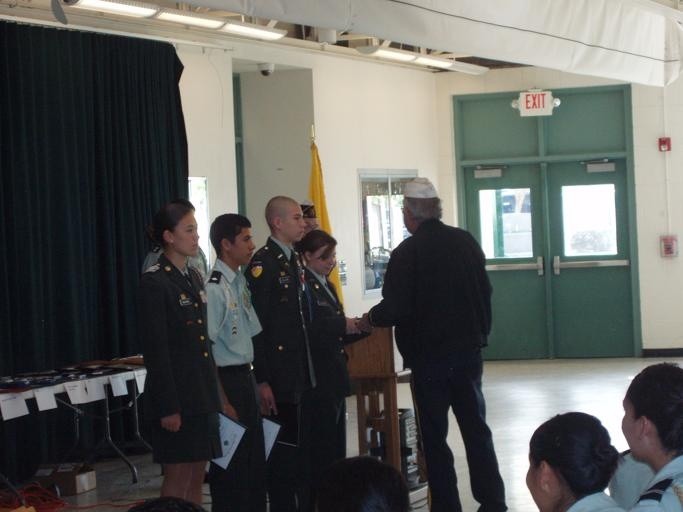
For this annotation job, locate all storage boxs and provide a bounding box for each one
[33,462,96,495]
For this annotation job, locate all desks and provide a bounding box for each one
[0,355,156,507]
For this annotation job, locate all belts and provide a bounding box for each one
[222,364,253,371]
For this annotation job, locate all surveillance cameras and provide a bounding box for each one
[257,63,276,77]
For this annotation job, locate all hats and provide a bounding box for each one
[403,176,436,200]
[300,204,318,220]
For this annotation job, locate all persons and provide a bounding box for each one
[141,200,208,276]
[299,204,320,236]
[610,448,656,511]
[125,495,206,512]
[293,230,361,463]
[526,410,625,511]
[319,454,412,512]
[620,363,683,512]
[205,213,266,512]
[140,204,224,505]
[244,197,316,512]
[355,178,506,512]
